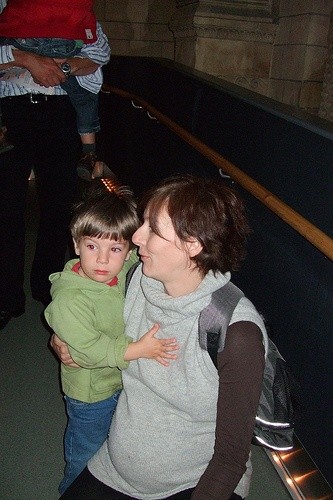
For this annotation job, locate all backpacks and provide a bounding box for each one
[124,260,296,451]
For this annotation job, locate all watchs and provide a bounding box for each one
[60,61,71,79]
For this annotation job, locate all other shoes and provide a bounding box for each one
[0,137,15,154]
[76,155,98,181]
[33,290,52,306]
[0,302,26,331]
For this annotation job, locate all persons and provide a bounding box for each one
[43,190,180,495]
[48,172,269,500]
[0,0,104,182]
[0,0,111,332]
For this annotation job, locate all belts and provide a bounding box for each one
[8,93,68,104]
